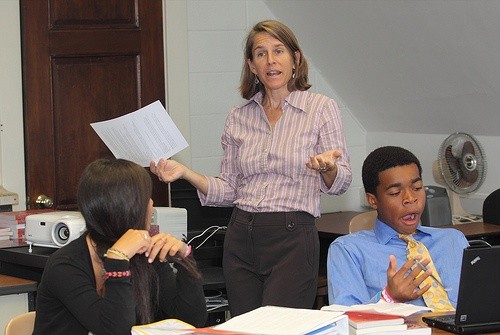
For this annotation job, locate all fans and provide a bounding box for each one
[431,130,489,225]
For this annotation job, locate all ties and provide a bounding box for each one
[397,230,456,312]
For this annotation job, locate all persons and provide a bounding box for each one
[31,158,207,334]
[149,20,351,319]
[326,145,471,313]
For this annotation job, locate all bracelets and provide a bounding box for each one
[100,270,131,280]
[104,246,130,262]
[181,245,189,261]
[382,287,400,303]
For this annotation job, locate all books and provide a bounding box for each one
[131,300,429,335]
[0,186,61,249]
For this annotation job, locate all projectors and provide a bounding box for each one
[24,210,87,253]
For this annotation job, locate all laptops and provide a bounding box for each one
[421,246,500,334]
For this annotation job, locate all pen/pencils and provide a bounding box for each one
[409,254,448,292]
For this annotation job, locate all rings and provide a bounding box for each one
[319,166,327,171]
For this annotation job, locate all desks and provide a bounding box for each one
[312,209,500,243]
